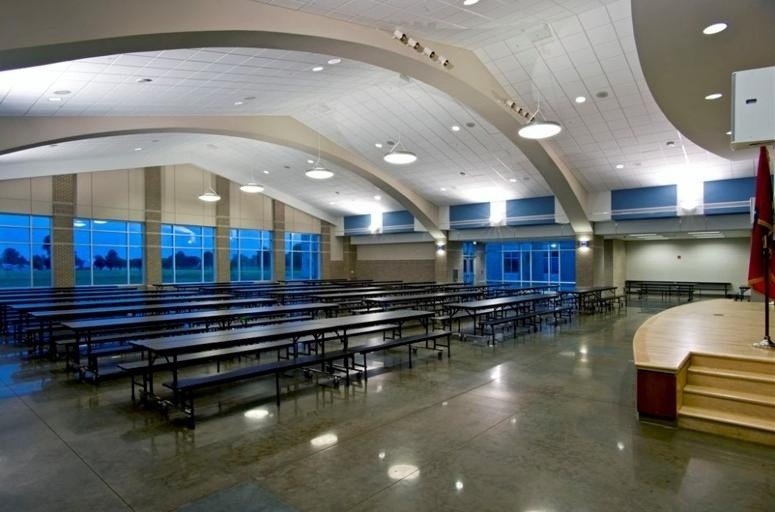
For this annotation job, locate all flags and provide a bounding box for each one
[747,146,775,306]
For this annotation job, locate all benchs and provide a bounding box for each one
[0,278,730,430]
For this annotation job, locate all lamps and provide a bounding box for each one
[516,86,562,139]
[384,78,416,164]
[392,30,449,67]
[304,104,334,179]
[196,171,221,204]
[506,99,534,122]
[239,171,263,192]
